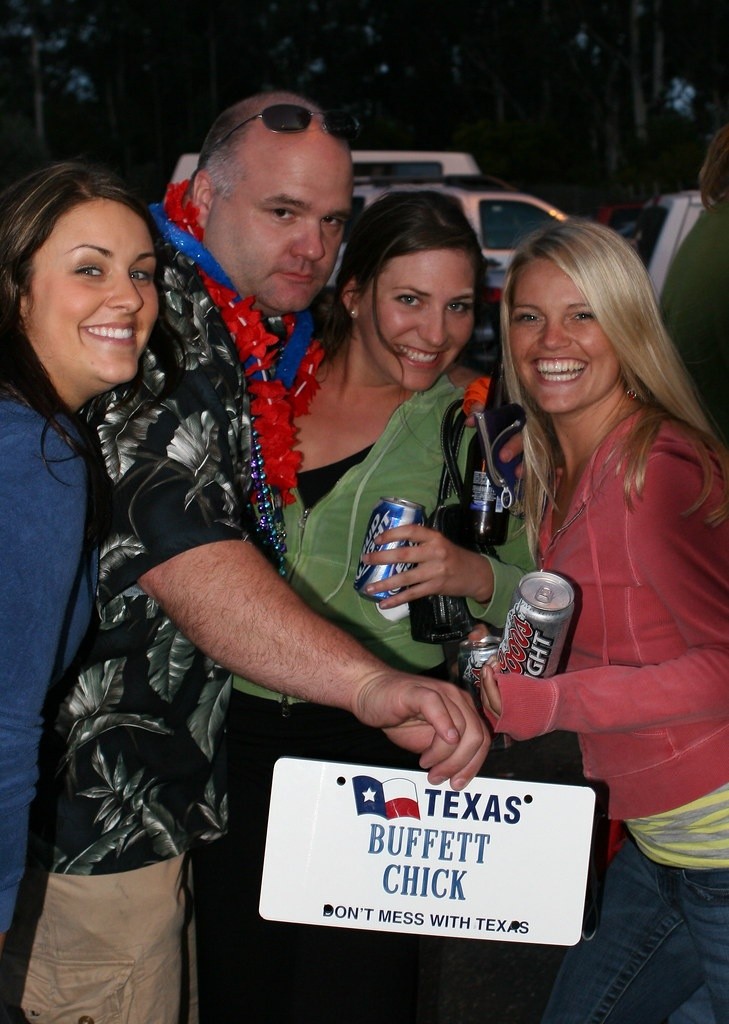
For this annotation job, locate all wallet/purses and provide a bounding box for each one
[474,403,534,518]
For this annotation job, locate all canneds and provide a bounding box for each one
[352,495,425,603]
[499,571,577,678]
[457,634,516,753]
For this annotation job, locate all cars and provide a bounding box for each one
[164,150,728,380]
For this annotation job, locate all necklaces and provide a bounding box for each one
[145,180,326,580]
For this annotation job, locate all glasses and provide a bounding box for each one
[214,103,361,148]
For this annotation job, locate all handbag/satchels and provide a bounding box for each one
[408,398,509,644]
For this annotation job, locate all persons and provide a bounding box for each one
[189,191,540,1024]
[0,91,525,1024]
[656,129,729,448]
[0,163,160,956]
[500,220,729,1024]
